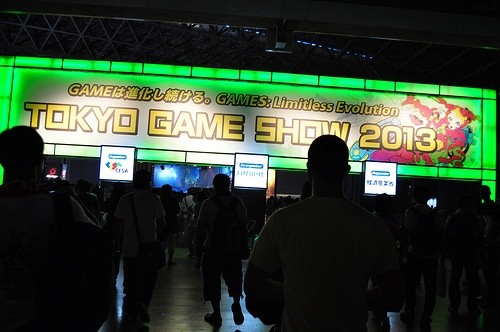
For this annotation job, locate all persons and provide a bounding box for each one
[180,188,214,267]
[367,183,500,332]
[196,174,248,326]
[158,185,182,267]
[75,179,100,225]
[243,135,405,332]
[0,126,110,332]
[265,180,313,219]
[115,171,167,323]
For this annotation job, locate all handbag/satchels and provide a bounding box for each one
[137,242,158,265]
[32,193,113,332]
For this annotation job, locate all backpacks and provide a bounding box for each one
[210,195,240,235]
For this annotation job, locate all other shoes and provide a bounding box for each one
[420,318,432,323]
[194,264,200,269]
[122,311,135,320]
[187,254,192,257]
[204,312,222,327]
[401,313,418,323]
[136,302,150,323]
[231,303,244,325]
[168,261,177,264]
